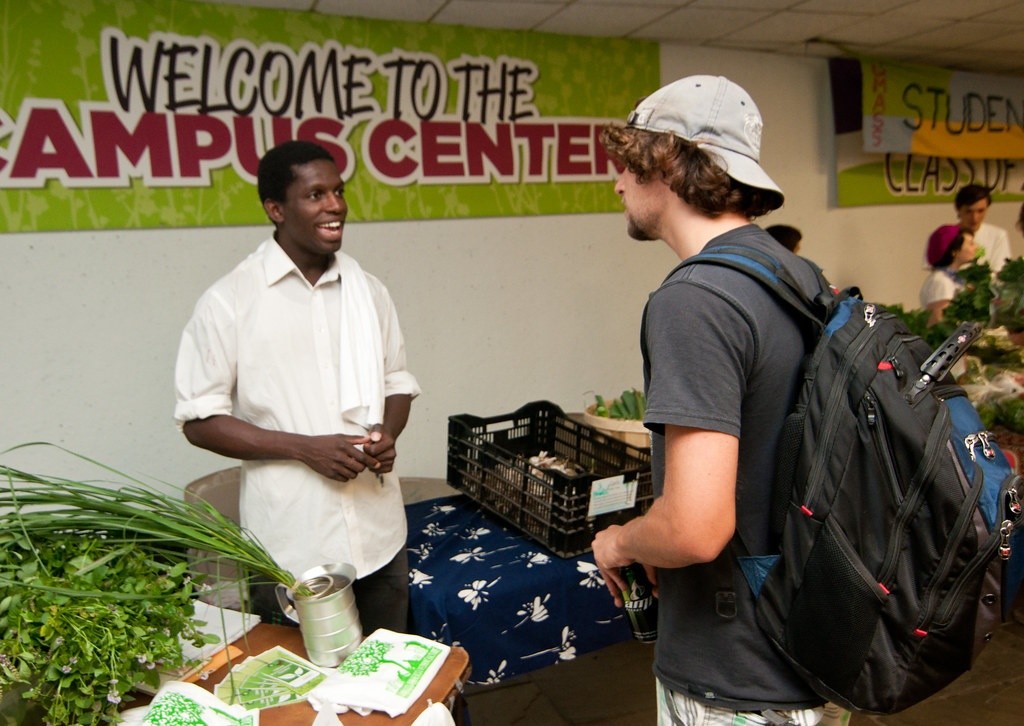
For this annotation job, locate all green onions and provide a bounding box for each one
[593,390,647,422]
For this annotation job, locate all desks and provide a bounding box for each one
[403,494,655,691]
[84,607,469,726]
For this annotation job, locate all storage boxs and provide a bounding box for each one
[447,398,653,557]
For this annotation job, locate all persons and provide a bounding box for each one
[173,140,422,638]
[766,225,802,255]
[954,183,1012,287]
[921,224,977,326]
[592,75,851,726]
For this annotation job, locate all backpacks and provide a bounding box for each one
[662,247,1022,715]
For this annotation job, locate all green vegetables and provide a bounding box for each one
[0,442,315,600]
[871,246,1024,433]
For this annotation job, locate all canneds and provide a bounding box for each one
[616,562,658,643]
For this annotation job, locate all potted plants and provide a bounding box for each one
[0,444,361,668]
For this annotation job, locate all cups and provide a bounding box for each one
[615,568,659,644]
[275,562,363,668]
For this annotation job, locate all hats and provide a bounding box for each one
[927,224,963,265]
[623,74,784,210]
